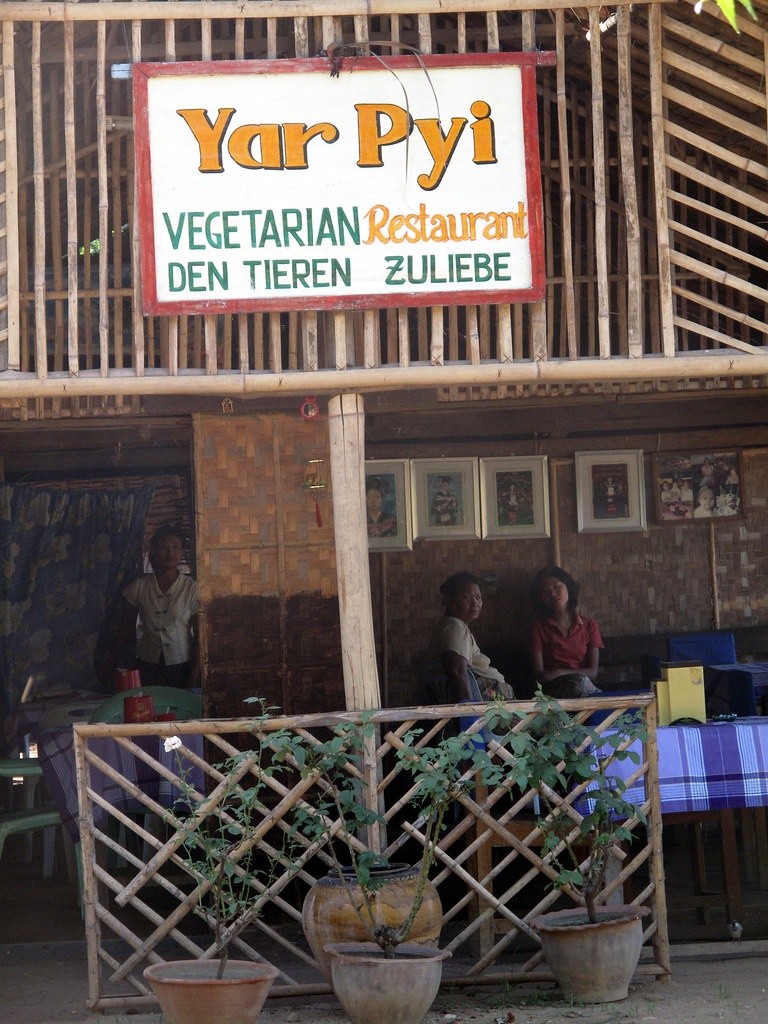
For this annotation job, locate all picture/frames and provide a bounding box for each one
[479,455,551,540]
[365,458,412,553]
[574,449,647,534]
[409,457,481,543]
[652,447,747,525]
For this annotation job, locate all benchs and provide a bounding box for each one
[484,625,768,694]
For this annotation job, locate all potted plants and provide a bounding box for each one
[486,681,658,1002]
[232,690,487,1024]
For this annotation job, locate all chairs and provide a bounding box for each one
[667,633,736,665]
[0,685,202,918]
[453,701,602,969]
[586,690,655,726]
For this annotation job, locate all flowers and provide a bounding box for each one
[162,697,310,978]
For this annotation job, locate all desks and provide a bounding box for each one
[566,715,768,937]
[708,661,768,716]
[36,719,202,920]
[3,695,109,759]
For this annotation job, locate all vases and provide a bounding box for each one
[143,958,280,1024]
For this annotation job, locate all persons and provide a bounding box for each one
[693,486,718,516]
[430,572,548,736]
[699,456,714,486]
[725,468,739,484]
[528,564,604,697]
[365,476,397,537]
[660,482,679,503]
[680,481,693,501]
[669,504,688,516]
[723,493,738,515]
[100,522,202,691]
[430,474,459,526]
[499,483,522,525]
[601,476,620,515]
